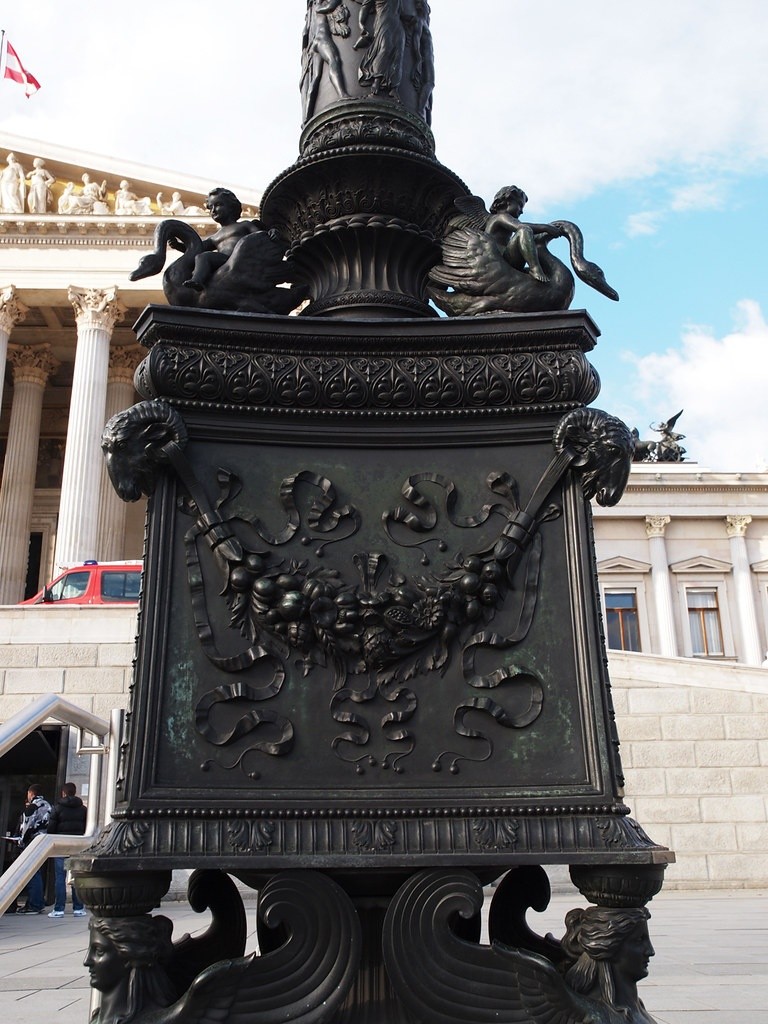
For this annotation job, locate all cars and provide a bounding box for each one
[17,560,142,606]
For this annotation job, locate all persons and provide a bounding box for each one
[487,183,563,282]
[114,180,156,216]
[24,158,54,226]
[157,191,206,215]
[0,151,27,226]
[83,917,176,1024]
[58,174,108,215]
[560,905,656,1024]
[165,186,282,289]
[13,783,52,914]
[44,782,89,917]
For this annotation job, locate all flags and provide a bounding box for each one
[2,38,42,98]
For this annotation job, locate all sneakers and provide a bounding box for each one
[16,907,44,915]
[73,909,86,917]
[47,909,64,918]
